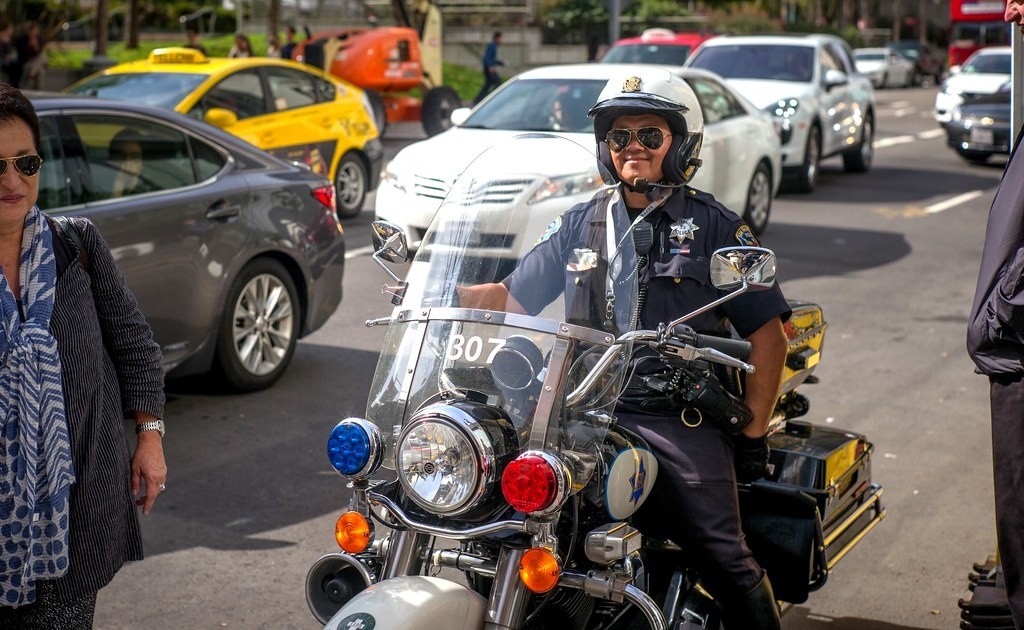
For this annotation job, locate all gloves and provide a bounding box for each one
[423,283,459,309]
[732,431,771,471]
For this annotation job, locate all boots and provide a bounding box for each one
[722,569,781,630]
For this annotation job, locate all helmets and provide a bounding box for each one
[587,69,703,182]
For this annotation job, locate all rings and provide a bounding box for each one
[160,484,165,491]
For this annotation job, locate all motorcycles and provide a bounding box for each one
[301,131,886,630]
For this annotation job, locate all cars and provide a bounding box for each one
[597,28,716,66]
[933,45,1011,129]
[946,80,1012,162]
[367,63,783,285]
[887,41,944,85]
[21,87,349,390]
[56,45,387,218]
[853,48,914,89]
[683,30,877,192]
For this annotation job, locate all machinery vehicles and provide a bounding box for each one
[289,1,463,135]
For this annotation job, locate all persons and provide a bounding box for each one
[269,26,297,59]
[473,31,504,105]
[92,129,150,200]
[0,21,45,89]
[966,124,1024,630]
[0,83,167,630]
[184,29,205,54]
[550,93,572,126]
[422,67,792,630]
[727,250,766,274]
[481,439,492,477]
[228,35,253,57]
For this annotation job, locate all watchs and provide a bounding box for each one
[135,420,165,437]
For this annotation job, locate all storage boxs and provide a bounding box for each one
[765,421,874,512]
[781,299,826,398]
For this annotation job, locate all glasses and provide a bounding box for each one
[605,126,672,153]
[0,153,44,177]
